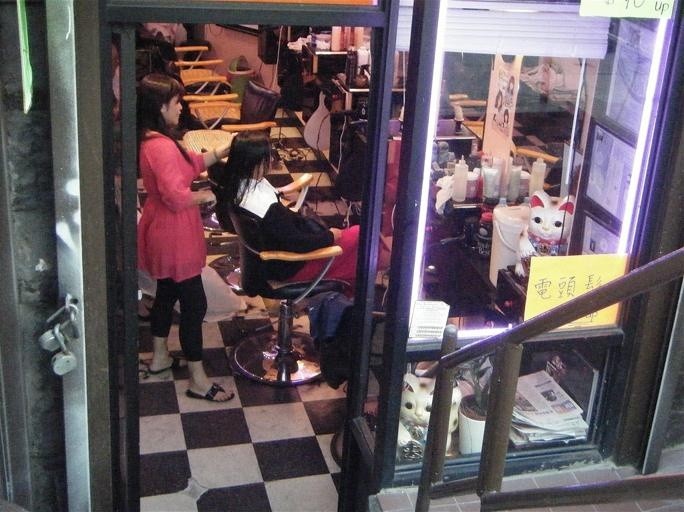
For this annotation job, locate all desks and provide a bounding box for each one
[485,267,528,330]
[436,118,477,162]
[348,119,402,184]
[433,187,482,244]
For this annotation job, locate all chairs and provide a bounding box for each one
[517,147,562,191]
[447,92,486,142]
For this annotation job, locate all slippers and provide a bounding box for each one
[186,381,234,403]
[149,355,188,373]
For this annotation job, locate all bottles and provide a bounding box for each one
[353,27,363,49]
[528,158,546,198]
[451,155,468,203]
[343,27,350,50]
[331,26,341,51]
[345,44,357,89]
[478,212,493,257]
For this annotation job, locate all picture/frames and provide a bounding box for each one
[582,117,637,227]
[591,16,658,144]
[579,211,620,254]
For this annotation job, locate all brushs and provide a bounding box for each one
[452,104,465,134]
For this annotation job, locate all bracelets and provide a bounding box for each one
[213,148,220,161]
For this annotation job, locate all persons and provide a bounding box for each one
[135,72,235,405]
[215,127,361,299]
[506,75,516,95]
[502,109,511,127]
[494,90,504,108]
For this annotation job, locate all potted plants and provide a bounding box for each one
[457,353,493,456]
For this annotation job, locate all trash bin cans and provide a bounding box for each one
[226,55,254,102]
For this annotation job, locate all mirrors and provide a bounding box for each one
[508,56,587,200]
[432,51,495,166]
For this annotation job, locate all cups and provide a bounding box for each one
[466,172,479,199]
